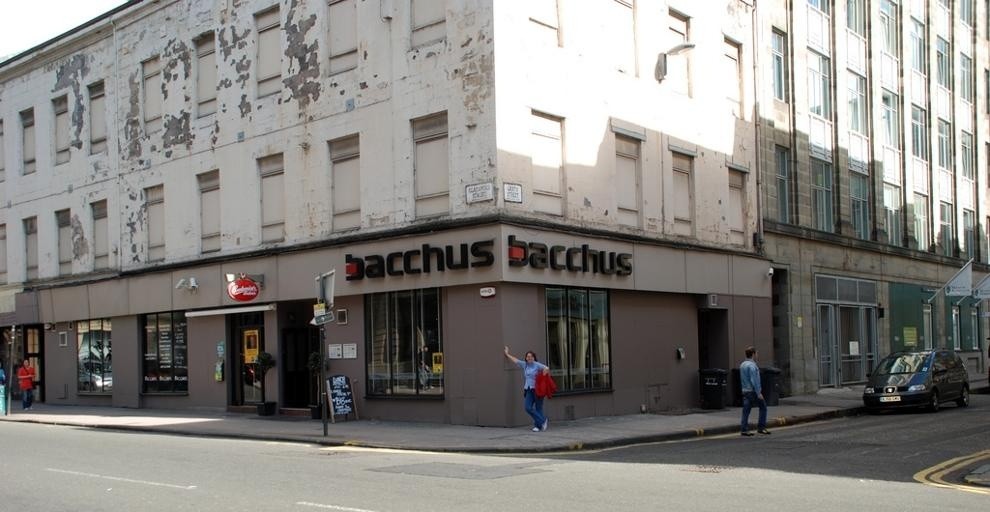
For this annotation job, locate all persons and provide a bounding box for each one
[502,344,553,432]
[0,362,6,385]
[737,345,773,436]
[16,358,36,410]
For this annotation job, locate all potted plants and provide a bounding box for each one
[307,351,331,419]
[253,351,277,416]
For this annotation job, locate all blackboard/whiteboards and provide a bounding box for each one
[327,375,357,415]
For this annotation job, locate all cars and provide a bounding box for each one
[863,348,970,414]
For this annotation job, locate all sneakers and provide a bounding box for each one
[542,418,547,431]
[24,406,34,411]
[758,429,771,434]
[741,433,754,436]
[533,427,539,431]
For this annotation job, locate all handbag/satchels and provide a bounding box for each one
[32,382,36,389]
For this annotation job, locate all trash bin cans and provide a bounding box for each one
[0,384,7,416]
[699,368,730,410]
[733,367,782,406]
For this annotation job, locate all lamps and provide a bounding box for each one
[658,44,695,76]
